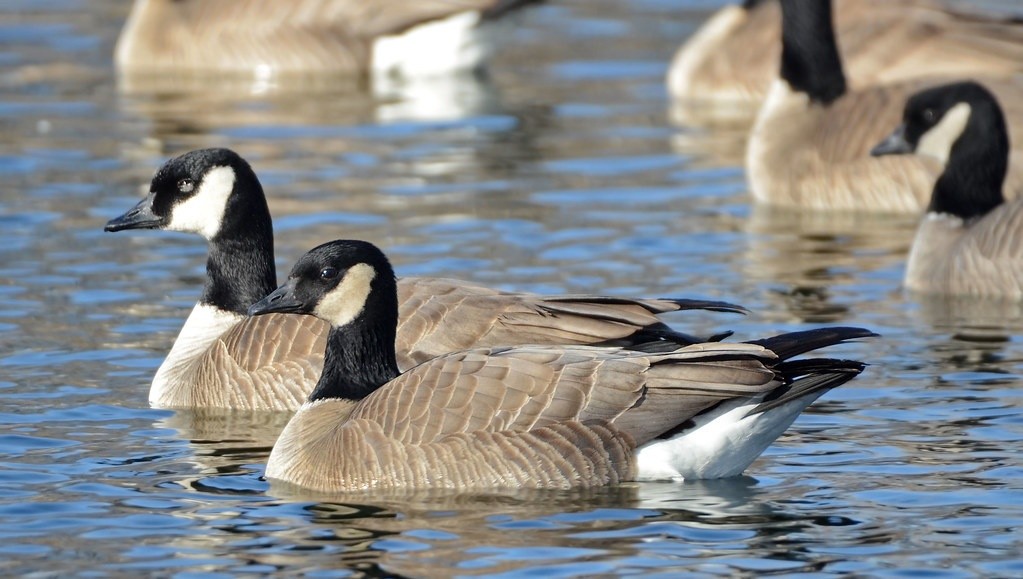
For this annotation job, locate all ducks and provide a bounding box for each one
[102,146,755,412]
[739,0,1022,216]
[870,81,1023,297]
[666,1,1023,129]
[113,0,558,75]
[247,236,882,489]
